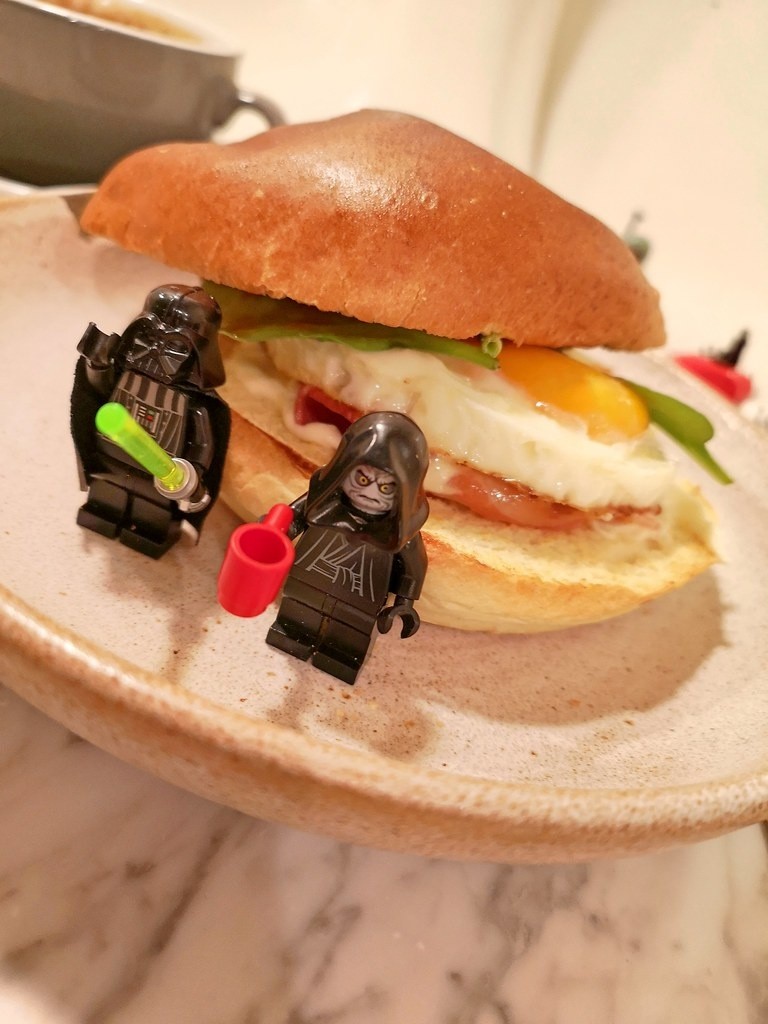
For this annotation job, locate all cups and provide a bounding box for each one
[0,0,288,186]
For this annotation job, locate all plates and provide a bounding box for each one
[0,180,768,864]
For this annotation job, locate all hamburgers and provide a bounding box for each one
[80,107,734,631]
